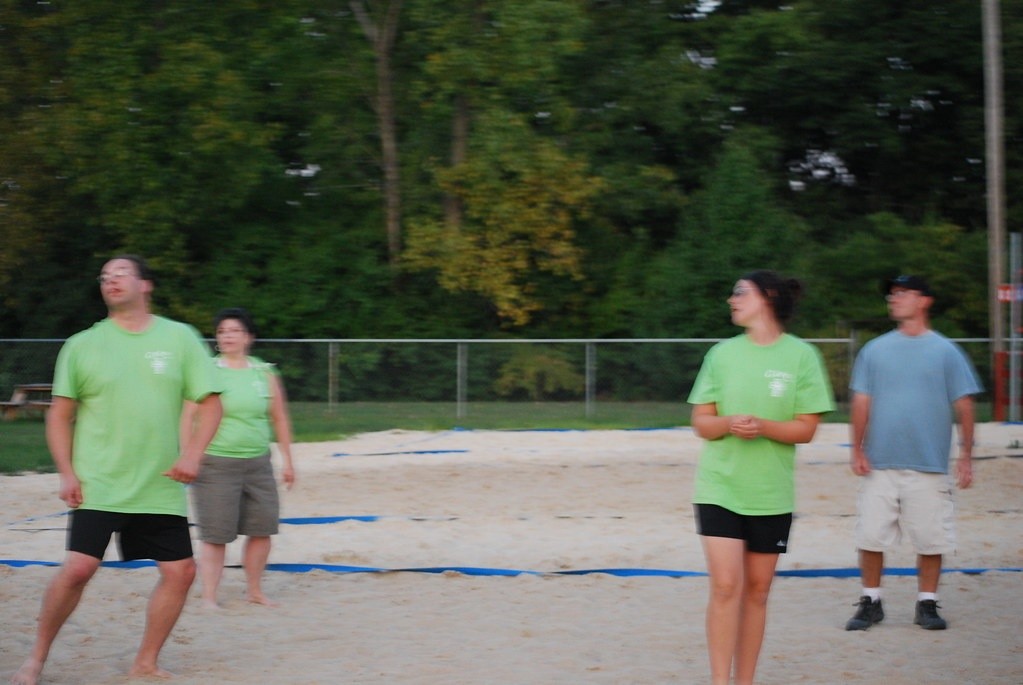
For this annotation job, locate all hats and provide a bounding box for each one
[880,274,934,297]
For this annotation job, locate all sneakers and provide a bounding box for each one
[913,598,947,630]
[845,595,884,630]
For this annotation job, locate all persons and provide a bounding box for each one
[9,257,226,685]
[844,275,986,631]
[180,307,294,608]
[687,271,837,685]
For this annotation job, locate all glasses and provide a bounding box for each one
[733,286,765,297]
[885,290,918,301]
[97,269,140,284]
[216,327,249,338]
